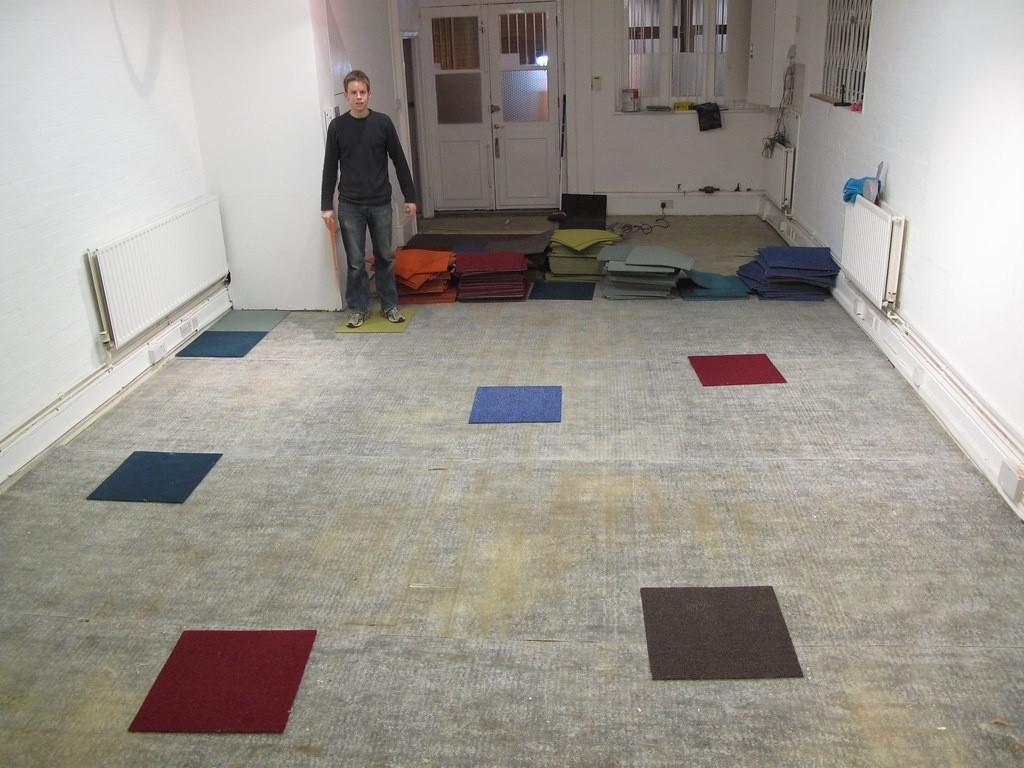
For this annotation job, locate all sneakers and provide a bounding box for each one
[385,307,405,322]
[346,312,370,327]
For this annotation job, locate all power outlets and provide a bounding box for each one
[659,199,674,210]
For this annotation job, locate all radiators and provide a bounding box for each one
[86,194,229,351]
[841,194,906,309]
[764,135,794,211]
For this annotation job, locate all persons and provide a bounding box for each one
[321,70,417,328]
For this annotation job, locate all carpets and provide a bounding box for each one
[641,586,803,680]
[174,308,292,359]
[334,309,416,333]
[86,451,222,504]
[530,229,838,300]
[127,630,317,734]
[366,249,529,304]
[687,354,787,387]
[468,386,563,424]
